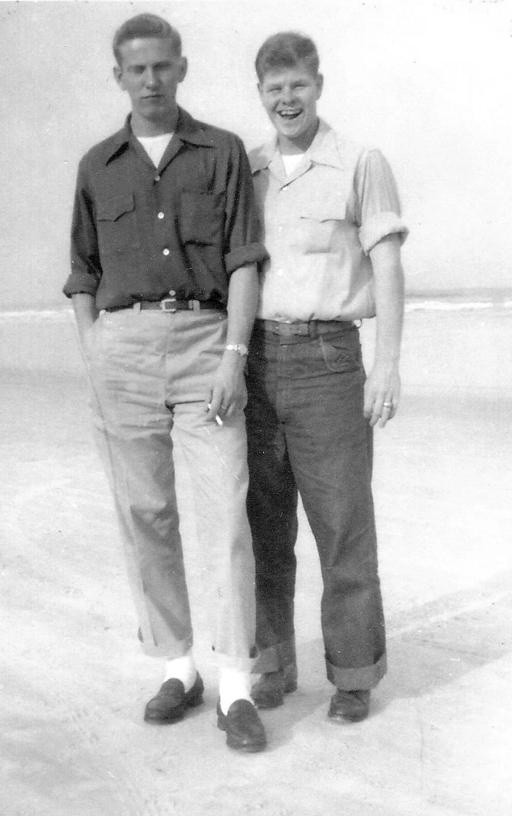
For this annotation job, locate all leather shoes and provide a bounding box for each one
[142,670,205,725]
[248,657,300,709]
[214,695,269,753]
[326,685,371,725]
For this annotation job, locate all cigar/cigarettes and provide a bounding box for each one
[208,403,223,427]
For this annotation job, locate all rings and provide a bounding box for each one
[240,407,244,411]
[383,403,392,407]
[220,405,226,410]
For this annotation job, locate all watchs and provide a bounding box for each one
[223,344,250,356]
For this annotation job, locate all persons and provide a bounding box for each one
[236,26,407,726]
[60,10,271,758]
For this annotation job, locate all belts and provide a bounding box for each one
[253,317,359,338]
[103,296,220,311]
